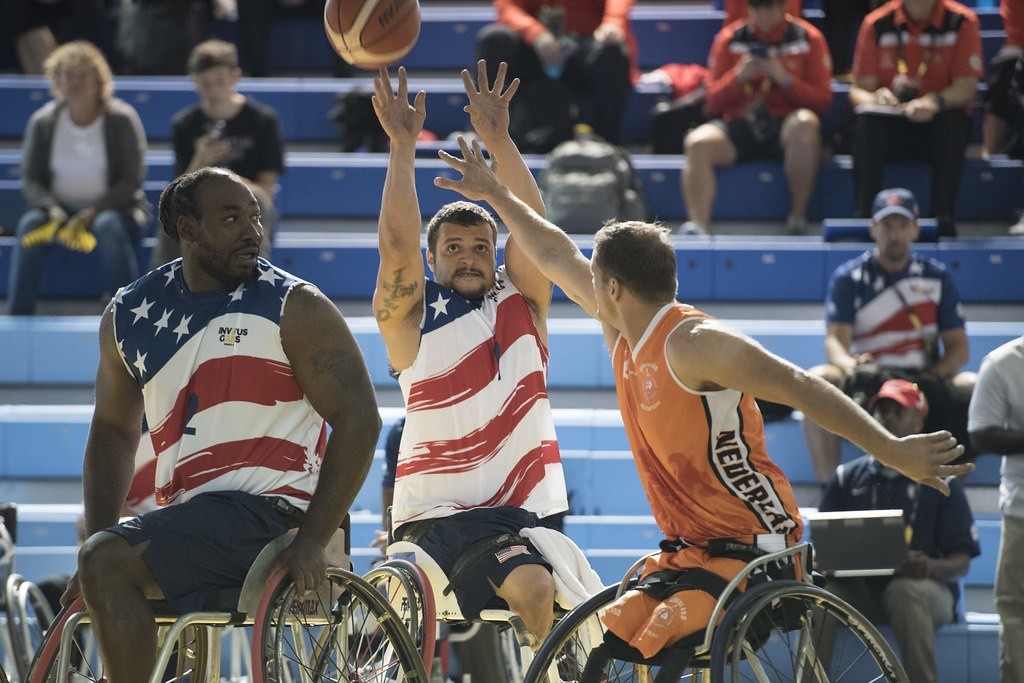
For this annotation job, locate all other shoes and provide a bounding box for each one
[932,211,956,238]
[785,215,810,230]
[678,220,705,236]
[1007,216,1024,236]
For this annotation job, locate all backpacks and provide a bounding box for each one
[542,123,645,233]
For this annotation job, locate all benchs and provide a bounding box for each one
[1,9,1024,683]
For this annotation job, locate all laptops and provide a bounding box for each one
[807,510,911,577]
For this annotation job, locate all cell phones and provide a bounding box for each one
[750,44,767,58]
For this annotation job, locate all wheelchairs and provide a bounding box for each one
[1,503,73,683]
[311,506,581,683]
[522,538,908,683]
[24,511,429,683]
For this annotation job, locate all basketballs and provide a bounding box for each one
[324,1,423,69]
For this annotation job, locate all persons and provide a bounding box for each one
[678,0,1024,245]
[5,42,156,318]
[60,166,383,683]
[801,186,977,492]
[371,59,568,651]
[793,379,981,683]
[149,40,282,264]
[433,136,973,660]
[0,0,353,78]
[28,507,137,672]
[967,333,1024,683]
[475,0,637,157]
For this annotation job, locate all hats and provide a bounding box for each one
[870,188,918,223]
[866,378,931,421]
